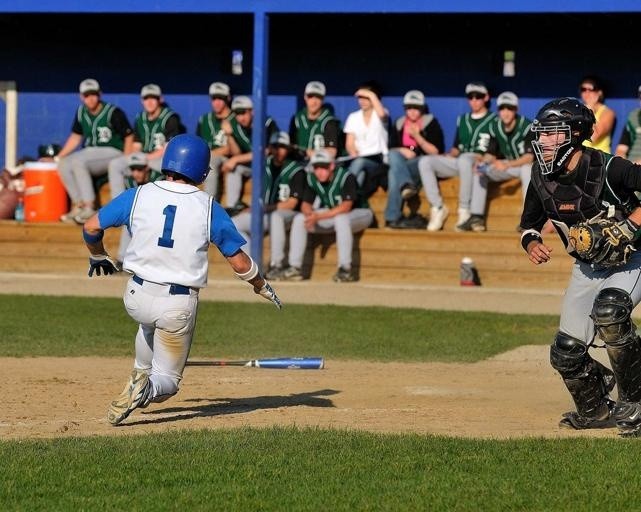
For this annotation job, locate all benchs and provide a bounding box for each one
[0,164,579,288]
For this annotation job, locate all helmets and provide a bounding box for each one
[161,133,209,184]
[531,98,595,175]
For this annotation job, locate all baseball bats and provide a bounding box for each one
[186,356,324,369]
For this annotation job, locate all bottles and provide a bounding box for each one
[460,256,475,285]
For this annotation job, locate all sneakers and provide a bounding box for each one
[385,215,411,228]
[427,205,447,231]
[274,264,303,280]
[333,265,353,281]
[264,268,284,279]
[108,369,149,425]
[559,416,616,431]
[400,183,415,198]
[59,206,93,224]
[470,214,485,232]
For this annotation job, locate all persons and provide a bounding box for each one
[80,134,282,425]
[580,77,615,155]
[615,84,641,165]
[515,97,641,436]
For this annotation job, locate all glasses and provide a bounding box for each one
[579,86,598,91]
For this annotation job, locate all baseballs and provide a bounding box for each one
[461,257,472,265]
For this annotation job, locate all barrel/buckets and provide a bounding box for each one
[22,160,67,221]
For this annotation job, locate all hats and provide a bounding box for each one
[80,80,99,96]
[310,151,333,164]
[210,83,231,98]
[269,131,289,148]
[466,84,488,93]
[230,96,251,113]
[497,91,518,109]
[402,90,425,106]
[141,83,161,99]
[304,80,326,97]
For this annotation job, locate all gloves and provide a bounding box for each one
[87,254,119,276]
[253,284,282,310]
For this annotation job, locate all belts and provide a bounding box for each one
[131,273,189,294]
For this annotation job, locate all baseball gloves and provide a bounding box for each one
[567,217,638,271]
